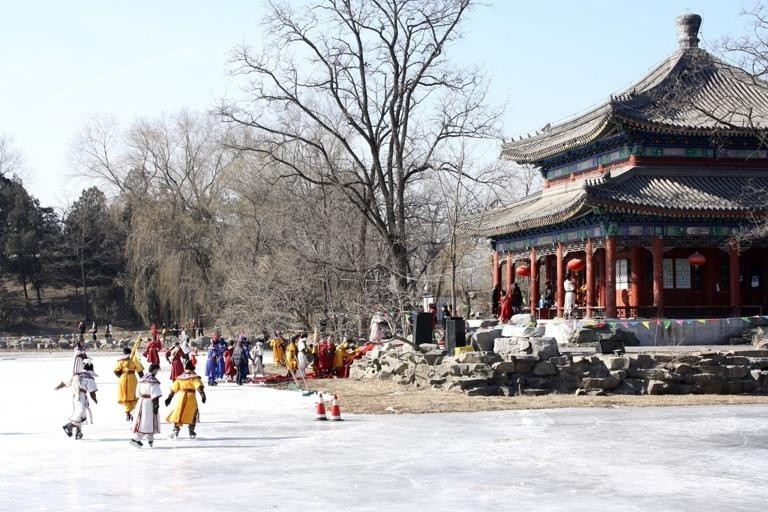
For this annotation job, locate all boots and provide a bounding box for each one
[76,429,83,437]
[189,427,196,435]
[66,423,74,435]
[171,426,179,438]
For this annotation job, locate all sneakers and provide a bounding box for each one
[208,376,256,385]
[132,439,143,446]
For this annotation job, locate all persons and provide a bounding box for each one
[62,320,357,446]
[564,273,578,319]
[498,290,512,323]
[370,303,453,342]
[510,283,523,313]
[544,281,552,308]
[538,295,544,308]
[492,284,500,317]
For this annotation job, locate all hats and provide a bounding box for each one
[185,361,195,370]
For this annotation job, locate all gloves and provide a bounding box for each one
[200,392,206,403]
[165,398,171,406]
[154,405,159,414]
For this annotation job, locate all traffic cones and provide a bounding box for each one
[314,392,329,421]
[329,393,343,421]
